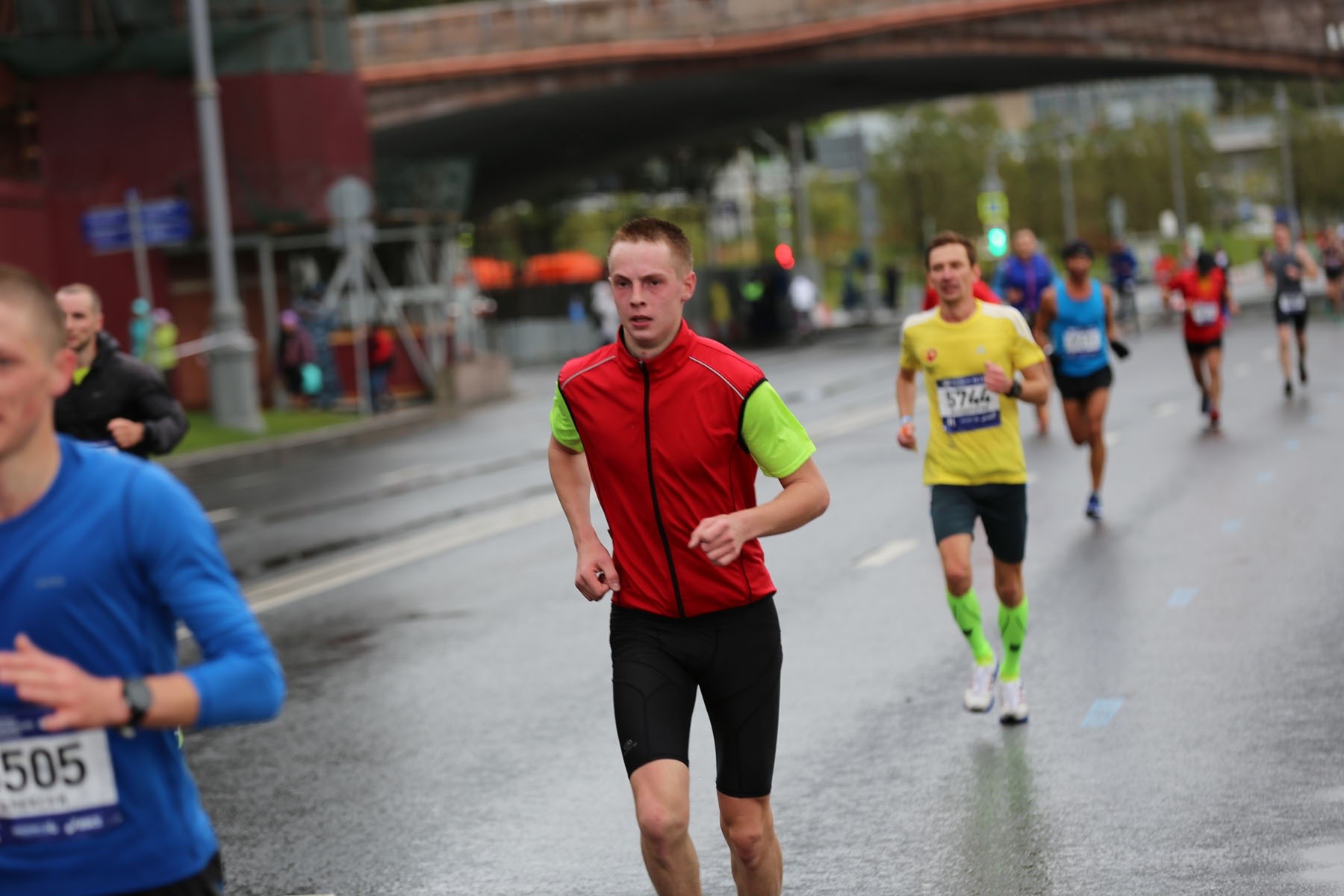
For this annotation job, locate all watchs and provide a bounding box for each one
[118,673,152,727]
[1006,378,1022,398]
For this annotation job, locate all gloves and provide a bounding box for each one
[1049,351,1064,370]
[1110,341,1129,357]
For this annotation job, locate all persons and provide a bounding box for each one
[897,229,1048,728]
[51,283,192,461]
[922,222,1344,519]
[0,264,285,896]
[131,295,395,413]
[549,218,828,896]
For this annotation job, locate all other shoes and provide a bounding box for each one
[962,651,1000,714]
[1086,493,1101,518]
[1201,389,1220,431]
[998,678,1030,724]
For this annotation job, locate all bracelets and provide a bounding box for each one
[899,416,914,425]
